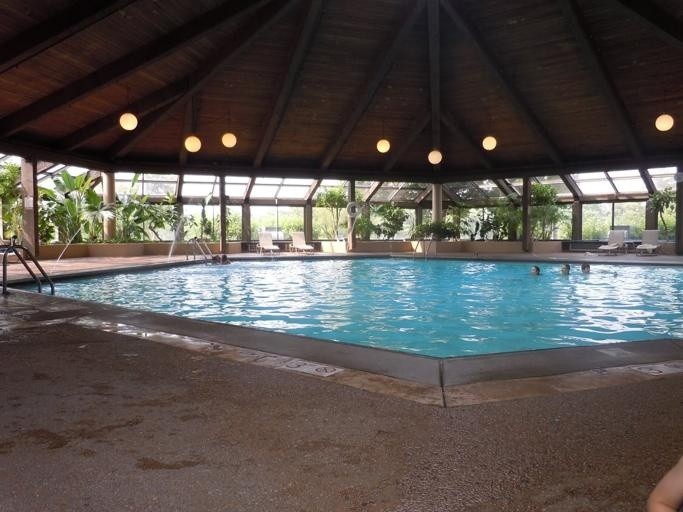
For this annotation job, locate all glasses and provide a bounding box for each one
[184,97,202,154]
[427,114,443,165]
[482,118,497,150]
[222,108,238,148]
[655,113,674,131]
[376,117,391,154]
[119,87,139,131]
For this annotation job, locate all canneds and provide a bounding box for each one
[346,202,361,217]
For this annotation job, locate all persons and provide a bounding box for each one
[530,266,540,275]
[562,264,570,274]
[581,263,590,273]
[644,456,683,512]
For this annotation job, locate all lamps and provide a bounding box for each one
[634,230,662,257]
[256,232,280,255]
[598,229,628,256]
[289,231,315,254]
